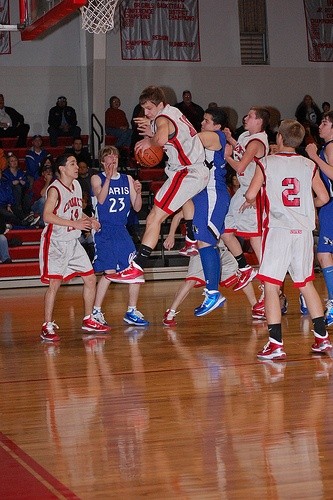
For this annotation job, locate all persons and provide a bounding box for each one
[47,96,81,148]
[0,135,98,265]
[38,154,110,340]
[104,86,333,361]
[0,94,30,148]
[90,146,149,326]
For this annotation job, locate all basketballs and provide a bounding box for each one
[134,141,163,168]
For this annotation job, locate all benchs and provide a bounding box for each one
[0,135,191,289]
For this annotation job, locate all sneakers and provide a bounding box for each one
[40,319,60,342]
[180,235,198,256]
[161,309,180,326]
[322,298,333,326]
[80,314,112,333]
[257,336,287,359]
[233,265,258,291]
[194,288,226,317]
[253,284,282,310]
[251,307,266,319]
[280,293,288,313]
[123,307,149,325]
[299,293,309,314]
[105,250,145,284]
[311,330,332,352]
[27,215,40,226]
[92,308,108,326]
